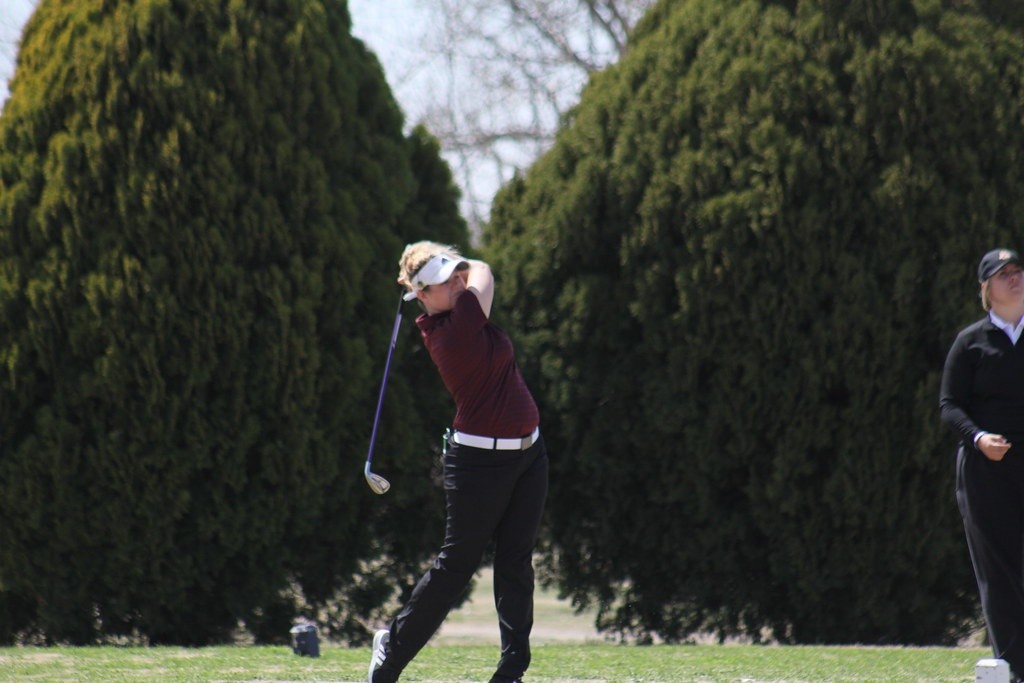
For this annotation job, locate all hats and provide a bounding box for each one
[978,248,1023,282]
[404,255,470,301]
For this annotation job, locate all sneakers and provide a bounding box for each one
[369,629,402,683]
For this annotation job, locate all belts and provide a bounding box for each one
[451,427,539,450]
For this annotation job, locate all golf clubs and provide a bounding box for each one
[363,286,410,495]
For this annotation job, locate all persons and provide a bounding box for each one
[369,239,542,683]
[939,246,1022,683]
